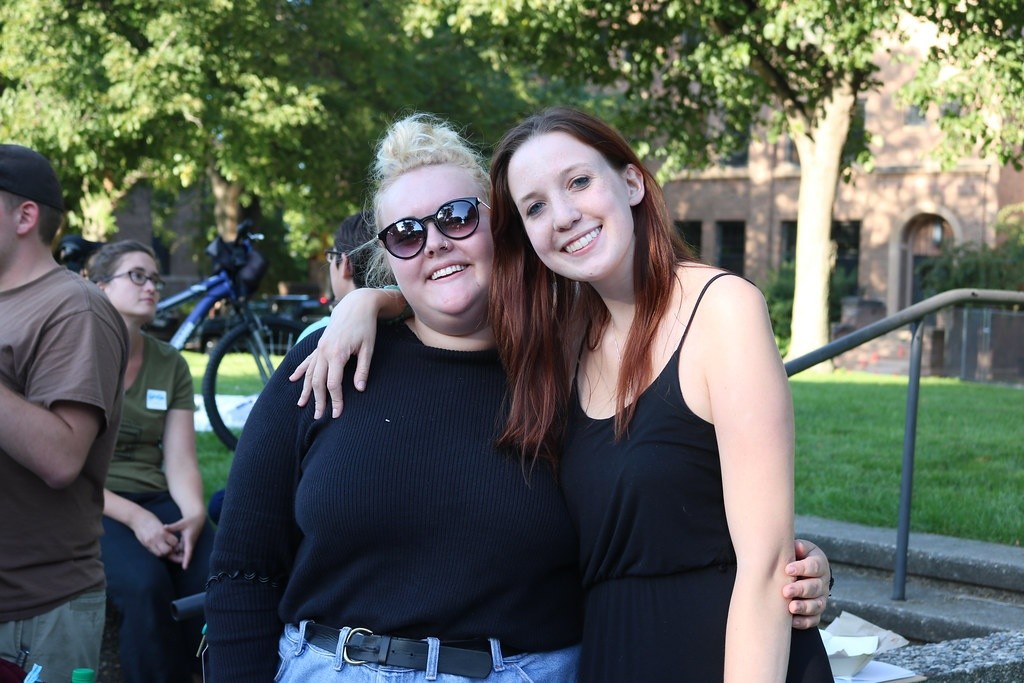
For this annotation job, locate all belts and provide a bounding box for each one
[304,624,531,679]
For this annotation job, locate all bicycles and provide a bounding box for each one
[52,216,329,453]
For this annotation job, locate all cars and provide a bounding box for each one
[198,293,338,355]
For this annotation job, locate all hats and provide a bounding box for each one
[0,144,65,212]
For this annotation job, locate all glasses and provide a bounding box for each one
[104,268,165,292]
[324,250,342,263]
[376,197,491,260]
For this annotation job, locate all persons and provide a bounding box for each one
[0,144,218,683]
[205,106,835,683]
